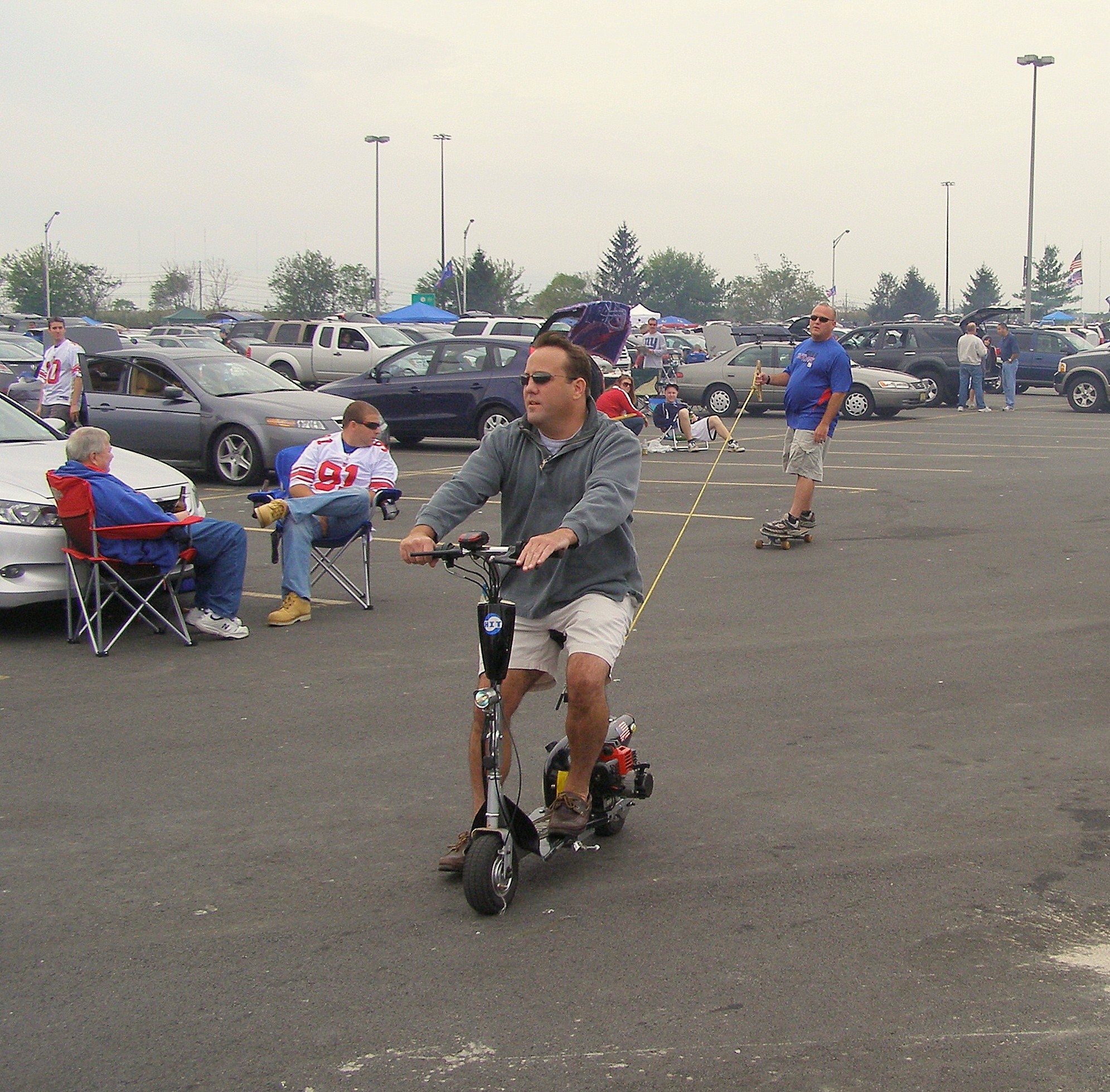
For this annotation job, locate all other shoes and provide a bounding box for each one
[548,790,593,837]
[966,400,976,407]
[438,830,472,872]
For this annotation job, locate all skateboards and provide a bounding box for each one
[754,527,813,550]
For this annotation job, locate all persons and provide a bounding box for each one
[35,316,86,433]
[55,426,249,638]
[399,330,643,869]
[255,400,398,626]
[219,328,228,345]
[591,316,746,454]
[26,321,38,339]
[866,315,1019,414]
[755,302,853,534]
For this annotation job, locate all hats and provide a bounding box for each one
[665,382,679,391]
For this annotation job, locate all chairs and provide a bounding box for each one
[46,470,203,657]
[249,444,402,610]
[439,356,486,371]
[649,398,709,451]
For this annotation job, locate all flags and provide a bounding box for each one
[1064,250,1084,290]
[434,260,453,288]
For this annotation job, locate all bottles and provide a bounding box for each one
[173,486,187,513]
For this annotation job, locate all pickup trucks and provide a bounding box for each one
[246,324,429,387]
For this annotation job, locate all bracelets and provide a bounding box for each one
[766,374,771,385]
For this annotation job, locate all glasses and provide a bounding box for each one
[621,382,632,388]
[811,315,833,323]
[520,371,577,385]
[349,419,380,430]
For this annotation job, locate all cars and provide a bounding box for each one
[0,393,207,610]
[0,309,929,488]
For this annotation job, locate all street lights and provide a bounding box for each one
[434,133,452,310]
[942,181,956,314]
[45,212,61,317]
[1016,54,1054,324]
[365,135,391,315]
[832,230,849,310]
[462,220,475,314]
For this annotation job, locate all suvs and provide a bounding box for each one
[834,308,1110,413]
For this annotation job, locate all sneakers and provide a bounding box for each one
[267,591,311,626]
[185,607,243,627]
[196,608,249,638]
[978,406,992,412]
[957,406,966,411]
[254,495,290,528]
[688,441,699,452]
[762,512,801,536]
[727,440,745,452]
[1002,405,1014,411]
[800,512,817,528]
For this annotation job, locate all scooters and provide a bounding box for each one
[409,530,653,917]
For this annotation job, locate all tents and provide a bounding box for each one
[376,301,460,323]
[162,307,206,323]
[629,302,694,324]
